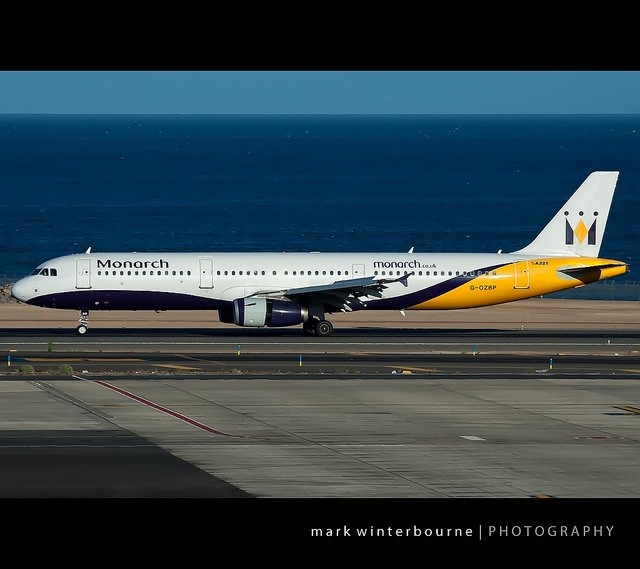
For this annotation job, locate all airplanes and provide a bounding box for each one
[12,172,630,334]
